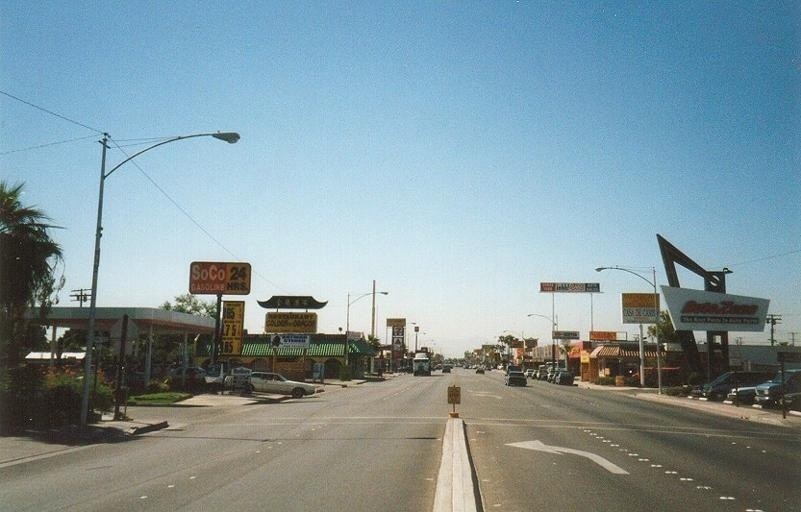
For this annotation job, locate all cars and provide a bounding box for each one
[700,368,800,413]
[167,366,207,381]
[245,372,318,398]
[497,363,574,387]
[435,361,488,375]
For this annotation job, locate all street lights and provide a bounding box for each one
[595,264,663,395]
[346,291,389,377]
[503,328,529,374]
[78,132,244,433]
[527,314,561,371]
